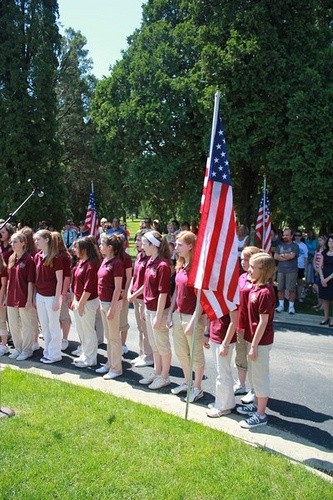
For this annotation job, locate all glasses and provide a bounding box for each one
[294,234,302,237]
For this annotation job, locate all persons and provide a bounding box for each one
[270,227,333,327]
[0,218,277,428]
[237,223,249,257]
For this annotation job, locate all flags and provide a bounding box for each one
[255,190,272,253]
[84,194,98,235]
[187,113,241,320]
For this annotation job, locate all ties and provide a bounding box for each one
[67,231,69,244]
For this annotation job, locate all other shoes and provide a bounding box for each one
[274,306,284,312]
[233,384,245,394]
[241,389,256,404]
[131,355,154,366]
[96,367,110,373]
[208,402,215,408]
[121,345,128,355]
[289,308,294,315]
[0,333,103,367]
[314,305,322,312]
[103,371,122,380]
[207,408,231,418]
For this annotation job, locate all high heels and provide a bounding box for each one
[320,318,330,325]
[329,323,333,327]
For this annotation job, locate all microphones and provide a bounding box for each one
[28,179,44,197]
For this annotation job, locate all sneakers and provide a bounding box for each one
[171,383,193,394]
[237,403,258,415]
[184,388,204,402]
[148,376,170,389]
[139,372,158,384]
[239,412,269,429]
